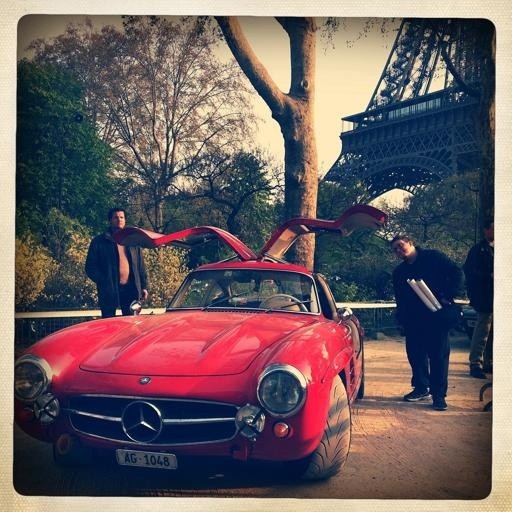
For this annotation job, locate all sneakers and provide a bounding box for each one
[432,392,447,410]
[404,386,431,402]
[470,366,485,378]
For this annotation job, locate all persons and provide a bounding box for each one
[464,216,494,379]
[84,206,149,320]
[390,236,464,410]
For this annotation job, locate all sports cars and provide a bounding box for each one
[14,205,389,481]
